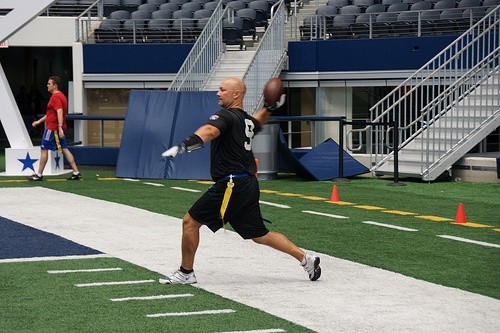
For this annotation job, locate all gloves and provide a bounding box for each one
[162,145,183,157]
[268,95,286,112]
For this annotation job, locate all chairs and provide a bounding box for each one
[42,0,500,52]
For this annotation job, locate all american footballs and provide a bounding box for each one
[263,77,283,105]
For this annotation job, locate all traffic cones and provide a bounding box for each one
[330,184,339,201]
[455,203,466,222]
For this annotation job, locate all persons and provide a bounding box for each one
[27,76,83,181]
[158,78,321,285]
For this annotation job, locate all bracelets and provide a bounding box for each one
[58,126,62,128]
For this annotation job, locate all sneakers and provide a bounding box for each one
[66,172,82,180]
[158,269,197,285]
[27,174,43,181]
[302,253,321,281]
